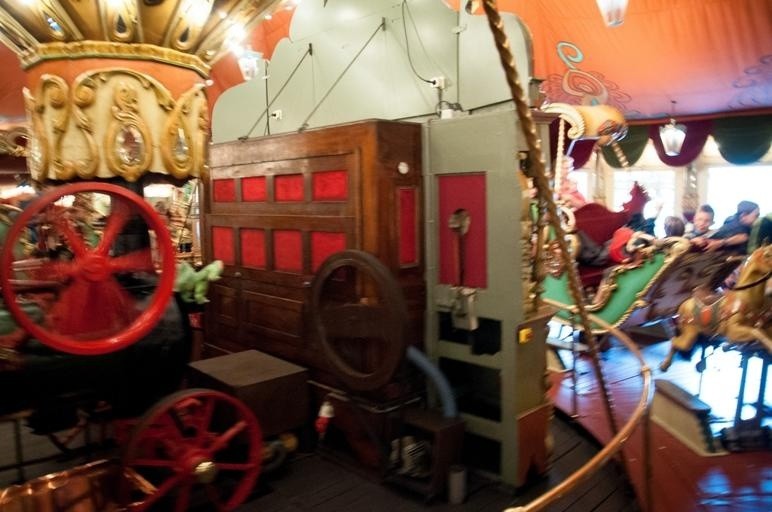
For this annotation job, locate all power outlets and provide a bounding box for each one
[430,76,447,93]
[269,109,284,122]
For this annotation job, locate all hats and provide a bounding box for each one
[737,200,758,215]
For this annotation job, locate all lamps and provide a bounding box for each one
[236,39,276,85]
[657,99,690,158]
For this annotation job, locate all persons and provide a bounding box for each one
[572,201,760,267]
